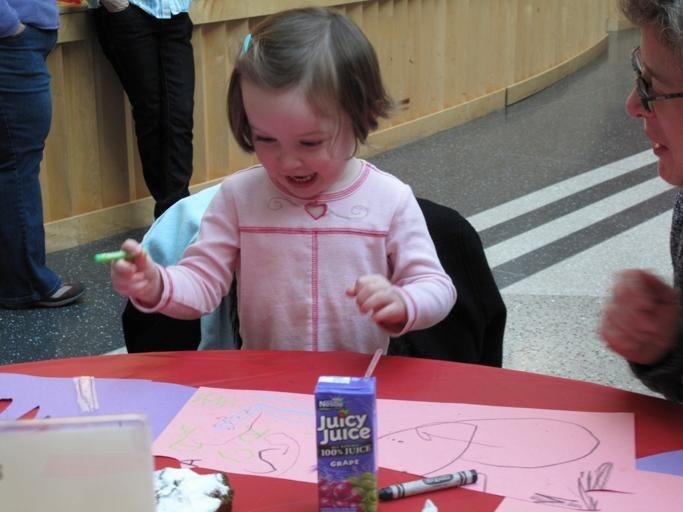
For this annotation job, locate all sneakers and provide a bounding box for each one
[3,279,89,309]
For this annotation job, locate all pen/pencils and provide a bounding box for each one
[93,243,146,264]
[377,470,478,501]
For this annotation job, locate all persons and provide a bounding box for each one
[0,0,88,311]
[82,0,195,220]
[107,5,460,355]
[594,0,681,406]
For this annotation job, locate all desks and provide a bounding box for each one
[1,350,681,510]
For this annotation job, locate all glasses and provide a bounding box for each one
[630,44,683,113]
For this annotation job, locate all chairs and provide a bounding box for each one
[119,196,507,370]
